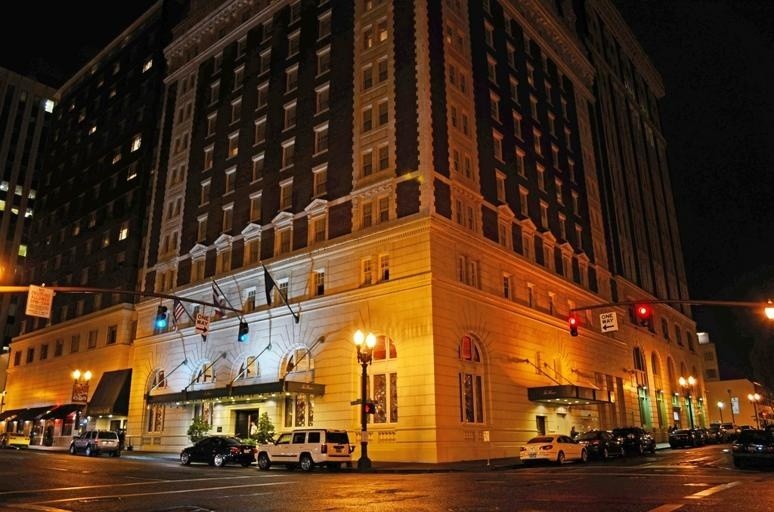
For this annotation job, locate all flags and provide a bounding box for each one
[172,297,185,333]
[212,284,226,320]
[263,265,275,305]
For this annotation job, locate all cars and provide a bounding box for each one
[669,423,756,449]
[731,430,774,471]
[0,432,30,448]
[520,428,656,466]
[180,429,255,472]
[70,431,119,456]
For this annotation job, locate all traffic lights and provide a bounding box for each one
[638,306,649,327]
[569,317,577,336]
[155,306,167,328]
[238,322,248,342]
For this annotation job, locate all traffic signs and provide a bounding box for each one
[599,312,618,333]
[195,313,210,336]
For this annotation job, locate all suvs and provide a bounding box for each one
[253,428,353,471]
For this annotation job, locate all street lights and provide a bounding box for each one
[354,330,376,468]
[679,376,694,428]
[73,370,91,430]
[718,400,723,423]
[747,393,760,430]
